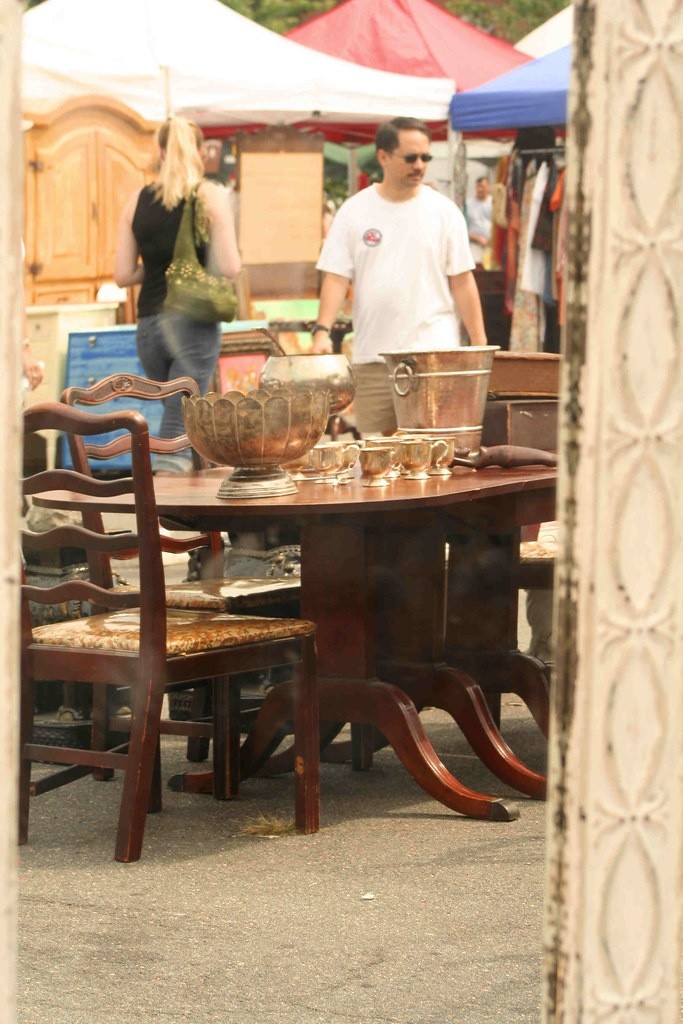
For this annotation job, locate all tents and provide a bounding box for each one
[195,0,534,218]
[448,43,571,217]
[20,0,456,142]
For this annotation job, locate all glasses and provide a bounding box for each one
[386,151,432,164]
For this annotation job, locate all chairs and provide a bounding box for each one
[21,373,323,865]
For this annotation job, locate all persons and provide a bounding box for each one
[310,117,489,365]
[461,176,494,264]
[116,117,242,462]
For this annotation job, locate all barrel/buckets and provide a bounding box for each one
[377,345,500,466]
[348,355,400,439]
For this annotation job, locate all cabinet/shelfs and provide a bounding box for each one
[22,97,167,329]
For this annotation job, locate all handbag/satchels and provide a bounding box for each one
[162,183,238,322]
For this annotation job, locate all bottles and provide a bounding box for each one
[20,363,46,405]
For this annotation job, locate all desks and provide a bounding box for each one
[27,458,559,821]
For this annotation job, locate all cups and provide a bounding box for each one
[360,436,403,487]
[400,434,456,480]
[306,444,360,484]
[326,440,363,483]
